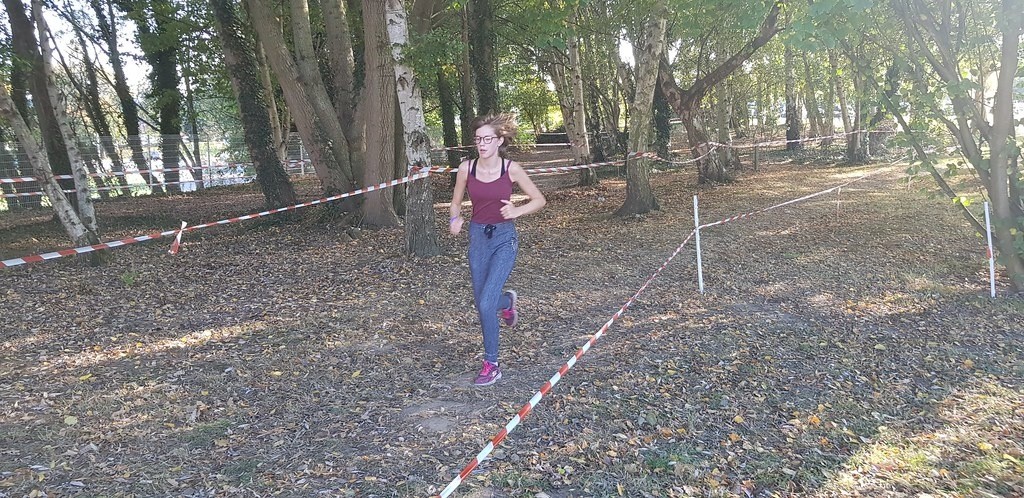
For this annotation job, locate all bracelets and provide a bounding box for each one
[450,214,458,223]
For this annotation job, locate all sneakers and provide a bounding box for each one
[474,361,502,386]
[502,290,518,327]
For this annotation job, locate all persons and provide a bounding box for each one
[450,110,547,385]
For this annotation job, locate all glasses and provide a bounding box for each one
[473,136,498,144]
